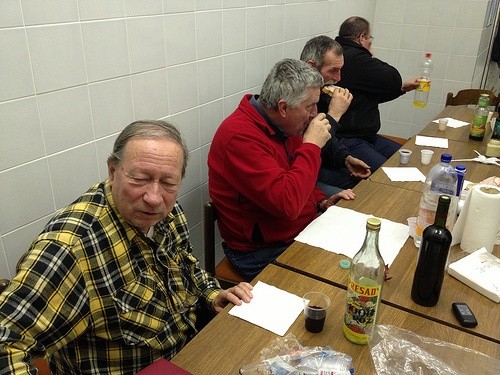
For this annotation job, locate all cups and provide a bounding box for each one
[420,149,434,164]
[302,290,331,333]
[438,118,449,131]
[399,149,412,164]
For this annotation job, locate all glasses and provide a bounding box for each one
[364,32,374,41]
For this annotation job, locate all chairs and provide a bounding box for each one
[203,199,251,290]
[443,88,500,107]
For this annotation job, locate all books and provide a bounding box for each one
[448,247,500,303]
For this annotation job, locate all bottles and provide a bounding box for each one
[411,193,453,305]
[469,93,489,141]
[343,216,385,346]
[413,52,433,108]
[414,152,458,249]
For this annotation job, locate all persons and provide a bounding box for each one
[297,35,372,203]
[206,57,355,286]
[311,17,422,174]
[1,118,255,374]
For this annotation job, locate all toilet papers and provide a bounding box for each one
[448,184,500,255]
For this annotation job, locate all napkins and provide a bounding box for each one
[447,245,500,304]
[382,165,427,184]
[433,116,471,129]
[228,279,311,336]
[415,135,448,148]
[294,203,411,272]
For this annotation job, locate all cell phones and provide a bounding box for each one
[452,302,477,327]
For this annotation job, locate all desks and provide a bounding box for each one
[275,179,499,342]
[367,134,499,198]
[165,263,500,375]
[412,104,500,143]
